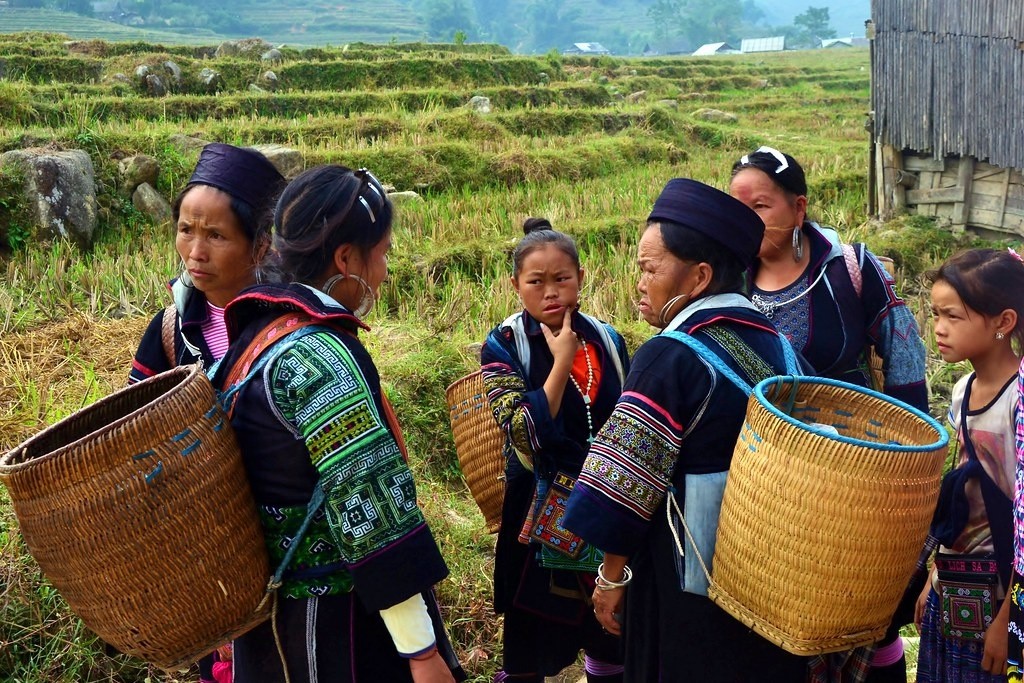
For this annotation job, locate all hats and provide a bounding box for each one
[186,143,287,210]
[646,177,766,272]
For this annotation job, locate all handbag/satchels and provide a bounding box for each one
[932,551,999,644]
[518,469,604,573]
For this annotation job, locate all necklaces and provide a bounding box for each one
[748,262,828,319]
[566,336,596,450]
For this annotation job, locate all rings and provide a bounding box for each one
[593,607,598,615]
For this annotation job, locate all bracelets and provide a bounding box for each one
[410,646,440,661]
[594,561,632,590]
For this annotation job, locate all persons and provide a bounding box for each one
[122,145,286,683]
[559,175,820,683]
[479,218,632,683]
[727,143,931,683]
[197,162,472,683]
[910,246,1024,683]
